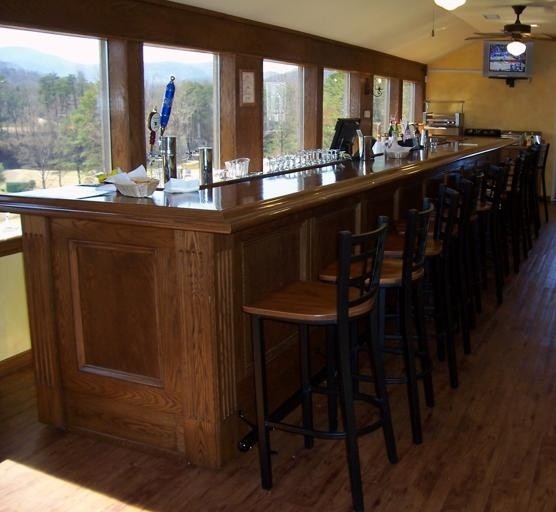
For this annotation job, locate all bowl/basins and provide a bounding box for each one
[112,176,159,198]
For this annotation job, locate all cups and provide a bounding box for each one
[225,147,341,178]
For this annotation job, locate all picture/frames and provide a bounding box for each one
[238,68,258,107]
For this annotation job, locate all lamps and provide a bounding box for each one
[463,5,555,58]
[365,75,386,97]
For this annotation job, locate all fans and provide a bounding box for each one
[506,40,527,57]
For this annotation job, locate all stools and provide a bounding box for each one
[369,177,474,392]
[318,198,437,444]
[241,215,400,511]
[425,142,550,330]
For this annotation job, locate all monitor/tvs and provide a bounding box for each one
[482,40,531,78]
[328,117,360,152]
